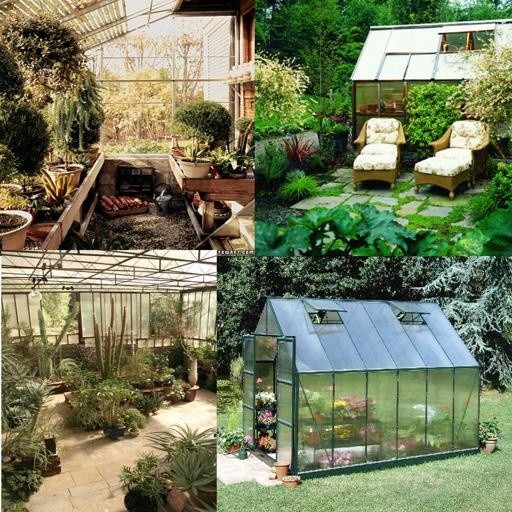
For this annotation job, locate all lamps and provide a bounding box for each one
[28,283,42,301]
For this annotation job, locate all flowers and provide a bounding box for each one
[255,377,383,471]
[219,428,245,448]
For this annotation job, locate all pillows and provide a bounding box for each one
[449,120,485,149]
[366,117,400,144]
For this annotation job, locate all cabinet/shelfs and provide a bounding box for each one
[168,154,255,241]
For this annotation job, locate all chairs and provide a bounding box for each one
[413,119,490,200]
[351,118,407,191]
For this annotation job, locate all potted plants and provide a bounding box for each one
[477,415,503,453]
[2,297,204,466]
[171,99,255,179]
[332,122,350,149]
[119,444,217,512]
[0,70,108,264]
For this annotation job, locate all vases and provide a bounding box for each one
[225,444,241,452]
[275,463,289,480]
[282,475,300,488]
